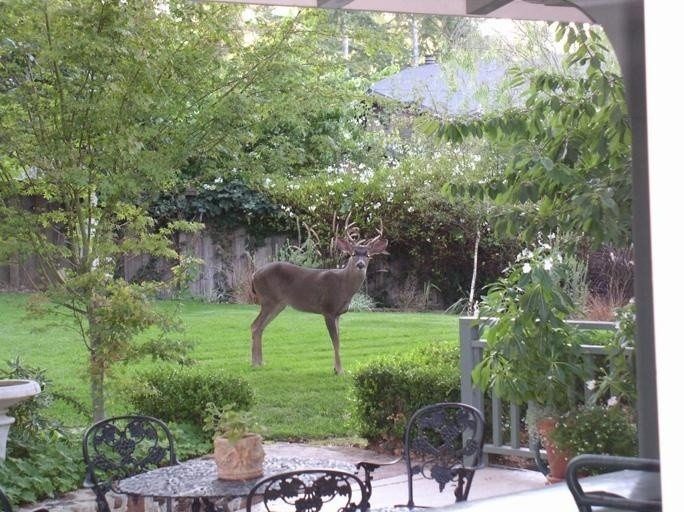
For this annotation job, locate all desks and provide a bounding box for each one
[115,456,360,507]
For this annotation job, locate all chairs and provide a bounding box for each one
[566,454,660,512]
[245,468,372,512]
[353,402,489,512]
[81,413,218,512]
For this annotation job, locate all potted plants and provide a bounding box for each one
[535,405,637,485]
[200,399,270,482]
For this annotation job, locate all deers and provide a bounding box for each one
[250,208,392,376]
